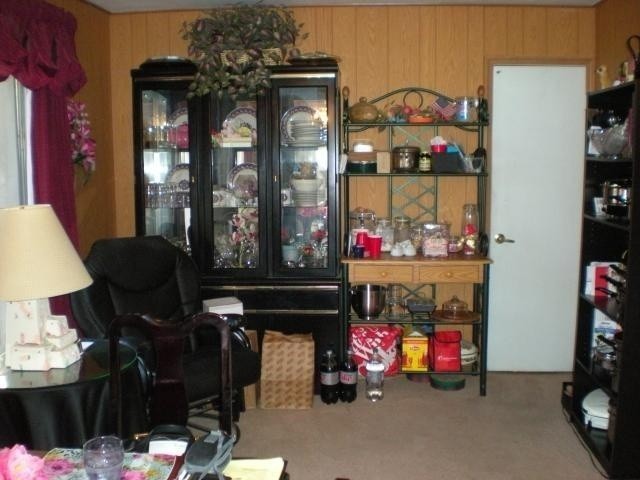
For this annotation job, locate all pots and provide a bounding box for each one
[348,284,388,322]
[598,176,632,209]
[593,331,621,394]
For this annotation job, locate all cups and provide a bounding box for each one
[82,434,125,480]
[353,231,384,260]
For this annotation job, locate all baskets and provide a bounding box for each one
[220,48,282,66]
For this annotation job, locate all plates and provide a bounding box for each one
[164,163,190,196]
[292,191,325,208]
[280,106,324,148]
[166,107,188,129]
[221,106,258,145]
[225,162,258,200]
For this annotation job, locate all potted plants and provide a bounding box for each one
[179,6,310,100]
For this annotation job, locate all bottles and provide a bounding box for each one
[455,95,474,123]
[319,349,358,405]
[462,203,480,236]
[349,206,376,233]
[365,348,385,404]
[374,216,451,260]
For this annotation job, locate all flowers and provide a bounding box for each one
[66,97,98,189]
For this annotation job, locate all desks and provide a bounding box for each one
[0,337,138,448]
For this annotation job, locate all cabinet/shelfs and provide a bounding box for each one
[561,60,640,480]
[340,86,493,398]
[130,58,343,393]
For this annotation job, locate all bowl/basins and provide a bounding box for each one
[289,178,321,191]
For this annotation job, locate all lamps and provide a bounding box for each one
[1,204,95,373]
